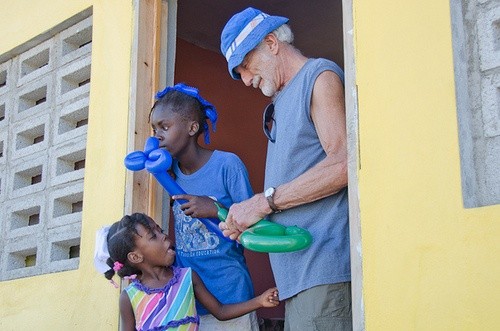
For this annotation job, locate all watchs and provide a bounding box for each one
[264,187,282,215]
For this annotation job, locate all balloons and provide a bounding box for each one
[214,202,312,254]
[126,137,240,248]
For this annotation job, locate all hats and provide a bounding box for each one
[218,8,293,82]
[154,83,218,146]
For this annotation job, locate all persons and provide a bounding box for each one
[219,8,353,331]
[149,85,261,331]
[101,214,279,331]
